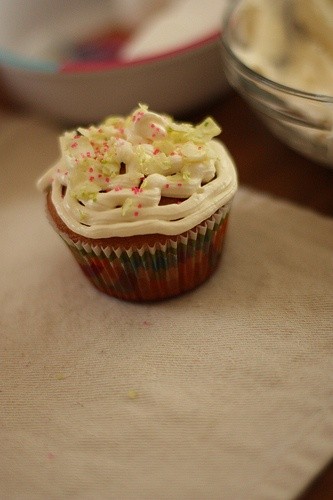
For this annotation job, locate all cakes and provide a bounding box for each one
[35,103,239,303]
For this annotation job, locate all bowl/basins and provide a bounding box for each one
[220,0,333,170]
[0,0,234,127]
